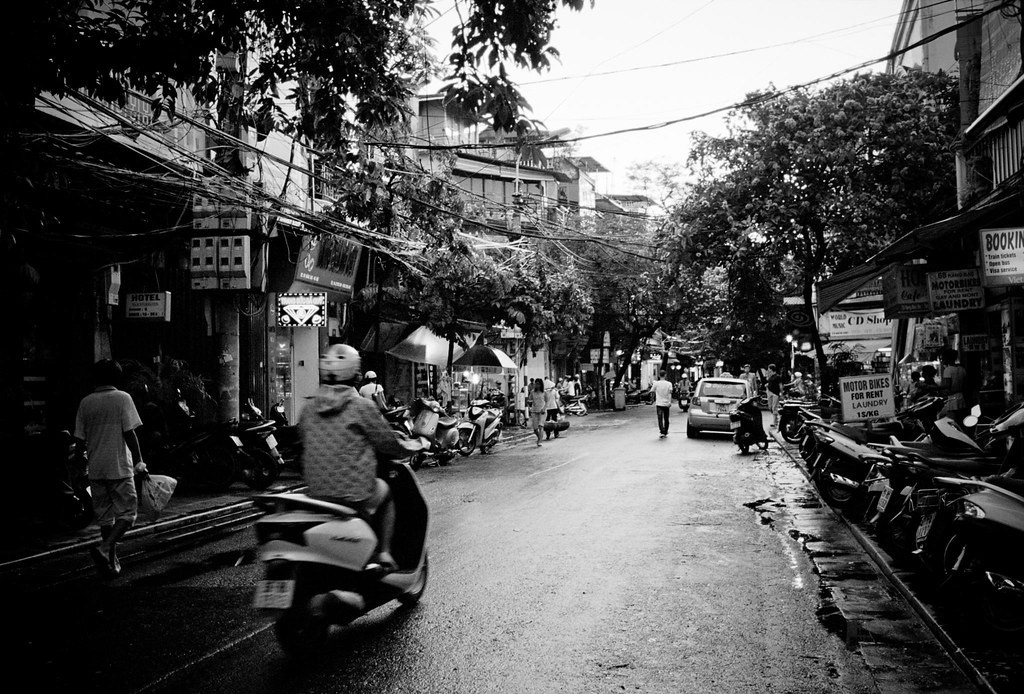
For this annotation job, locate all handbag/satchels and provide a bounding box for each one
[134,462,177,522]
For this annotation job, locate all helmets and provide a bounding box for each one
[364,371,377,379]
[682,374,688,378]
[320,344,360,383]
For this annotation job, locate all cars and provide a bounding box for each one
[687,372,751,439]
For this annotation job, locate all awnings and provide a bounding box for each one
[815,190,1009,318]
[866,191,1024,265]
[803,339,892,365]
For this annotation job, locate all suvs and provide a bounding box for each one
[628,389,650,401]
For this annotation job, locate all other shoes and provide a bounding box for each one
[376,551,398,572]
[660,434,667,438]
[538,443,542,445]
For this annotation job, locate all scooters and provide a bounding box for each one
[729,394,769,455]
[675,383,692,412]
[243,431,434,661]
[384,393,504,472]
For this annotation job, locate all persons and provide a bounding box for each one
[299,344,411,571]
[915,348,966,397]
[544,380,559,440]
[765,364,782,427]
[359,371,387,410]
[527,374,582,417]
[675,373,693,398]
[783,368,814,399]
[739,364,758,406]
[906,371,923,398]
[911,365,939,402]
[649,370,673,438]
[529,378,548,446]
[74,359,151,574]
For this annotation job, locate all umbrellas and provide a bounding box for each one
[452,344,518,400]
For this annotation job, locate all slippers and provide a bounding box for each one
[91,547,116,577]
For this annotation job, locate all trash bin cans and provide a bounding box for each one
[614,388,626,411]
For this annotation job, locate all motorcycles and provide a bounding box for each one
[143,386,303,498]
[558,389,588,417]
[780,386,1024,593]
